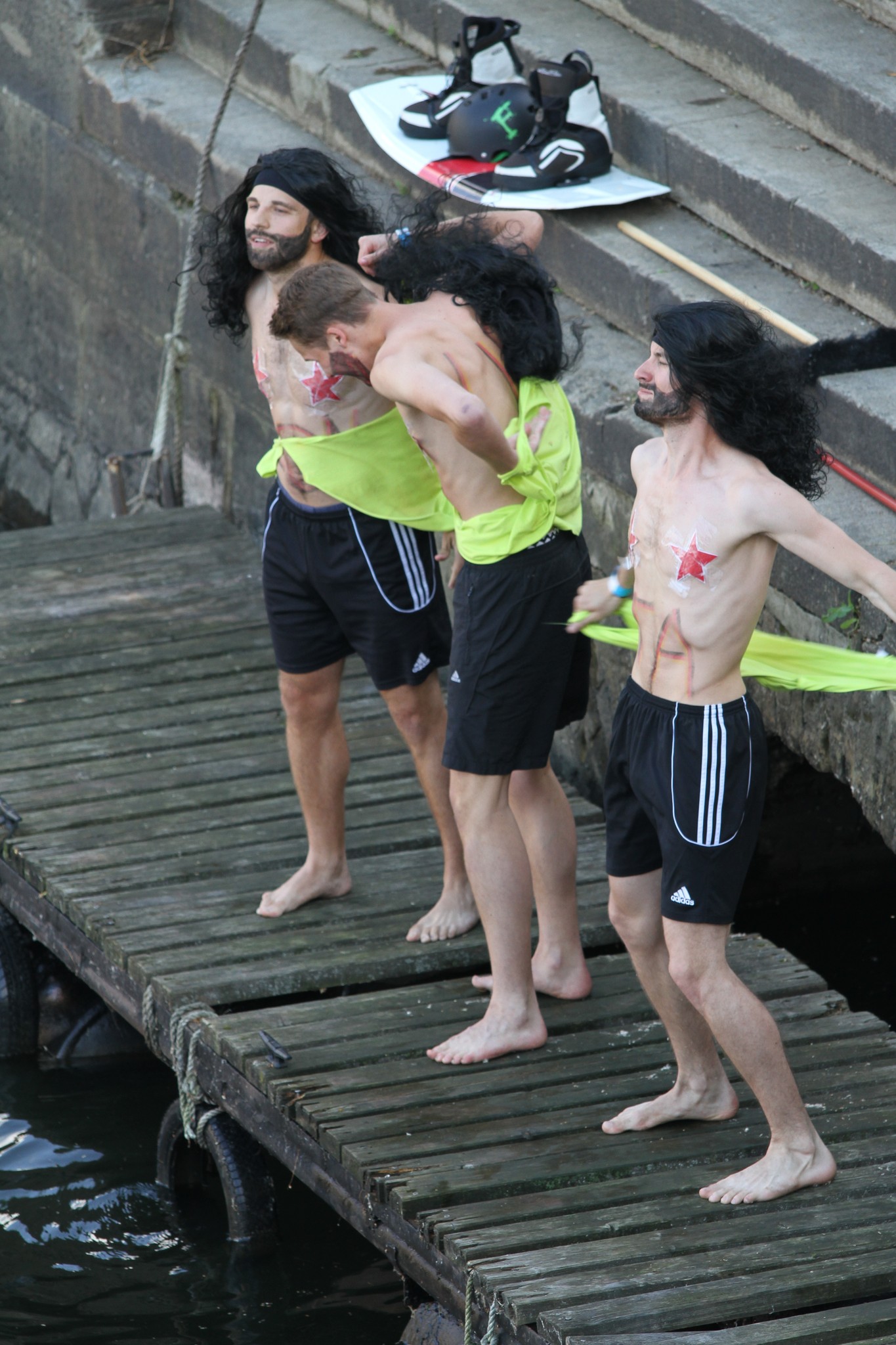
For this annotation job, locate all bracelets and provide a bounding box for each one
[606,570,632,599]
[394,226,412,248]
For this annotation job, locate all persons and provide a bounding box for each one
[172,147,479,944]
[267,209,593,1064]
[564,300,896,1204]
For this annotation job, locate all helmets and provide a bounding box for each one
[448,83,542,161]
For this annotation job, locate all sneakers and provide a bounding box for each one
[399,15,524,138]
[492,49,613,190]
